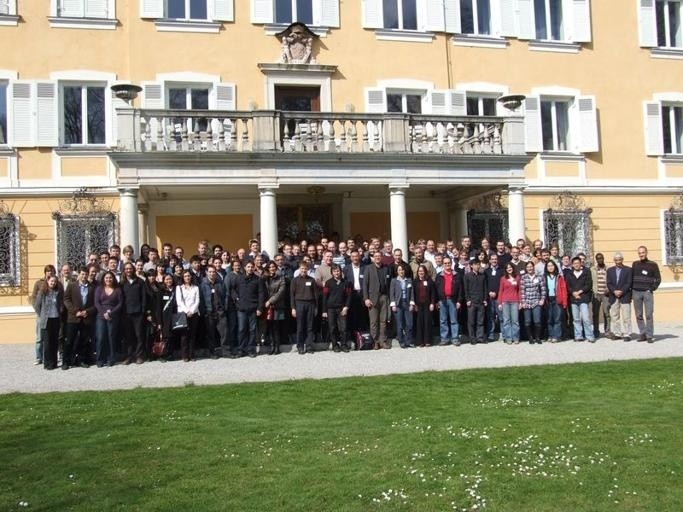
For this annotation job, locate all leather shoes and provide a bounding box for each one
[298,342,391,354]
[34,357,143,370]
[611,334,652,343]
[400,337,559,348]
[235,347,280,357]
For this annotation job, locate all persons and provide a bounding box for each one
[32,231,632,372]
[632,247,661,343]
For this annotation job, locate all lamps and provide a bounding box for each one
[306,186,325,205]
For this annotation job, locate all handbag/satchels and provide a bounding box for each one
[172,312,188,330]
[152,342,167,356]
